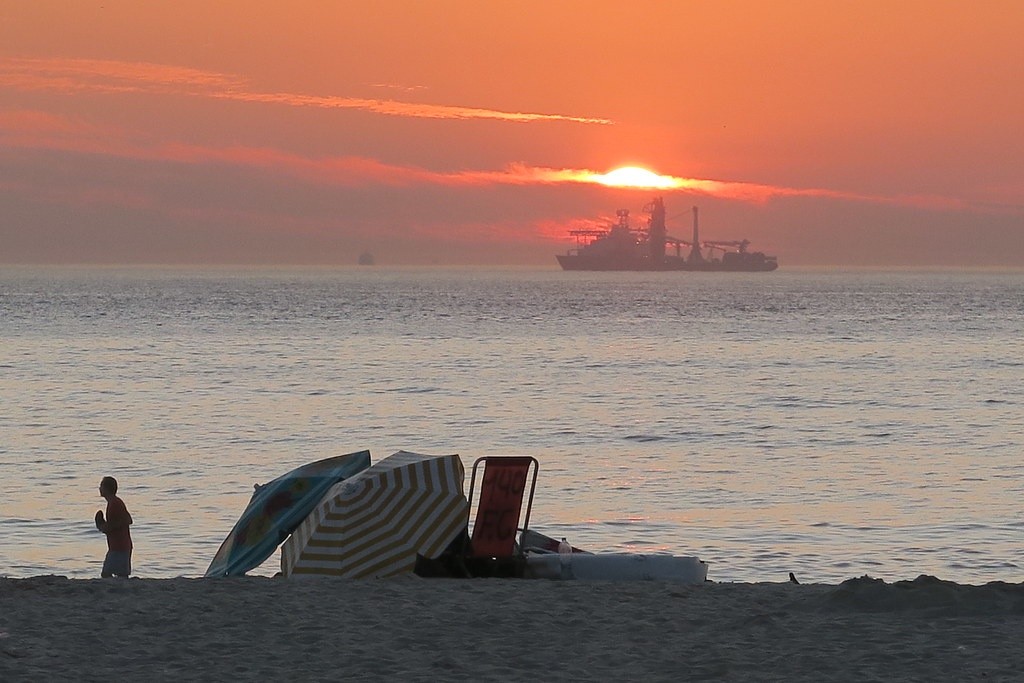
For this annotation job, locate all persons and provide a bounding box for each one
[95,477,133,577]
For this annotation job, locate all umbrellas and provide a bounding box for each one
[280,450,468,578]
[203,449,371,578]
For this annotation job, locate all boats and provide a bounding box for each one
[554,197,778,271]
[513,528,708,583]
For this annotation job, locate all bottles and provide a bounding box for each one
[558,538,572,555]
